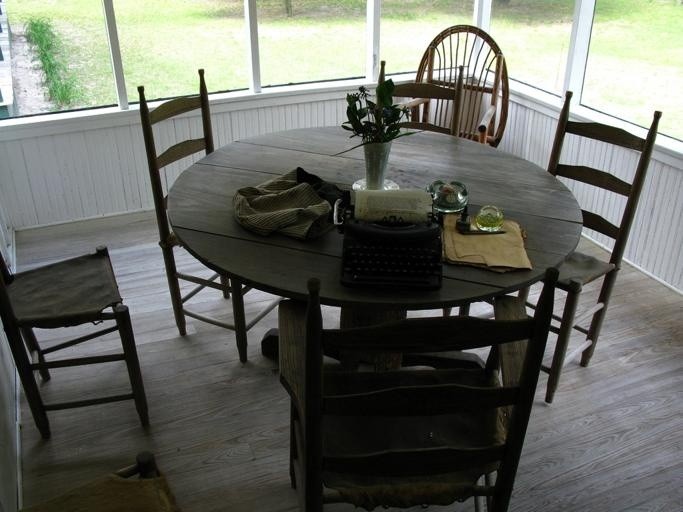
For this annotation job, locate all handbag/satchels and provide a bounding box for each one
[339,217,442,294]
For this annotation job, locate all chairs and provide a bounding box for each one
[457,90,662,402]
[136,69,295,365]
[378,23,511,146]
[20,449,184,510]
[1,246,150,440]
[272,267,560,512]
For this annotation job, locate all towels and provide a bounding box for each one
[439,211,534,272]
[231,165,351,244]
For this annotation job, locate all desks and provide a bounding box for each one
[167,123,583,367]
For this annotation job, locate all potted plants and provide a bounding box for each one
[341,78,424,187]
